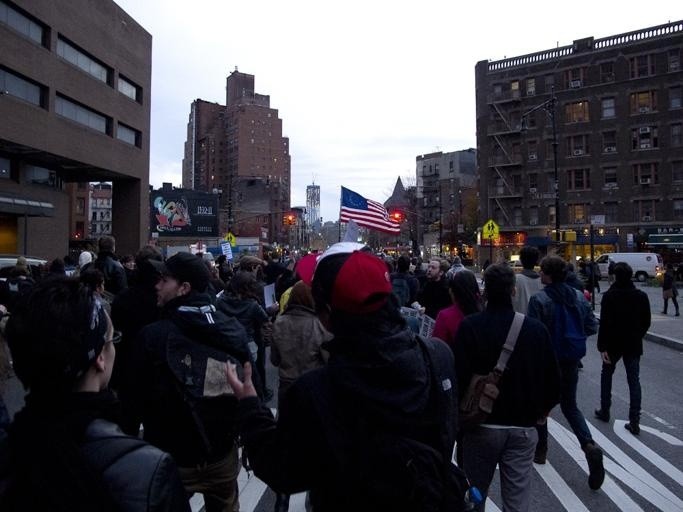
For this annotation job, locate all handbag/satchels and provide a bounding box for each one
[461,373,500,433]
[663,288,673,298]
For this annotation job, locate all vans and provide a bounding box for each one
[594,251,663,282]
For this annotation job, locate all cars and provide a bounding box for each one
[203,251,217,267]
[507,259,543,274]
[0,258,48,277]
[376,251,396,258]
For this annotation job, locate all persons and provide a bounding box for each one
[0,234,683,511]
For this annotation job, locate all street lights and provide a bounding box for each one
[211,183,234,234]
[518,84,562,252]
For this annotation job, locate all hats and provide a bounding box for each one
[297,241,392,313]
[148,252,211,291]
[240,255,262,265]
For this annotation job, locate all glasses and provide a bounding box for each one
[107,330,122,343]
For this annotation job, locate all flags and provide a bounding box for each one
[338,185,400,236]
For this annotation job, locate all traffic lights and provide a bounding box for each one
[287,215,292,225]
[291,215,295,225]
[394,211,400,220]
[282,216,287,225]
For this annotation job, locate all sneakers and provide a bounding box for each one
[625,423,640,434]
[583,440,604,490]
[594,408,611,423]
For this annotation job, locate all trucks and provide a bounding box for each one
[190,244,207,256]
